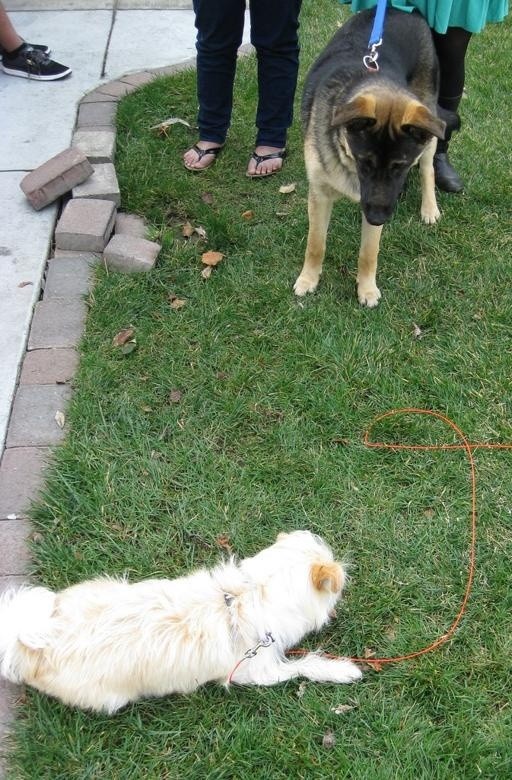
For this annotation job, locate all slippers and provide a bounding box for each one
[183,139,227,172]
[245,143,288,178]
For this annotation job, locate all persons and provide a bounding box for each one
[1,0,73,81]
[179,0,301,177]
[342,1,508,194]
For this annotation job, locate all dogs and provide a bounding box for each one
[292,5,447,309]
[0,530,363,716]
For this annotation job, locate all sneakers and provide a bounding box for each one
[0,43,74,82]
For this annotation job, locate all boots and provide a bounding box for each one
[432,93,466,195]
